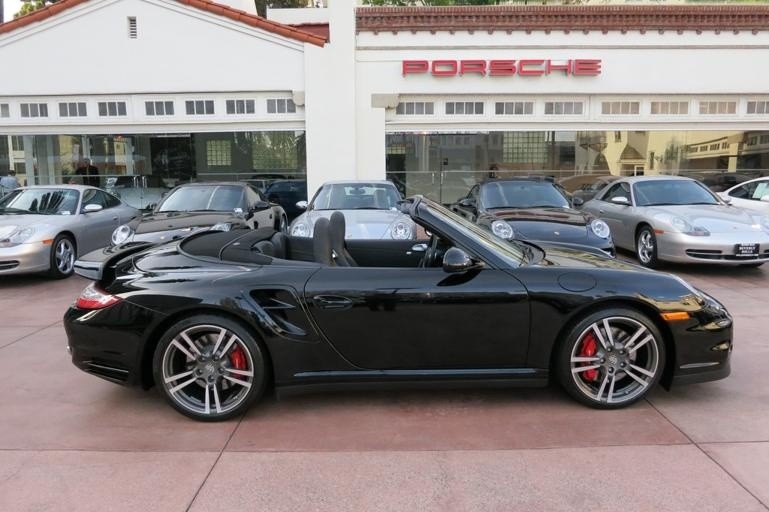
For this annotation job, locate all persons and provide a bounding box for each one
[75,159,101,188]
[0,170,19,195]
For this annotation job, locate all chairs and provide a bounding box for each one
[328,211,360,267]
[253,242,276,260]
[271,231,295,262]
[313,217,342,268]
